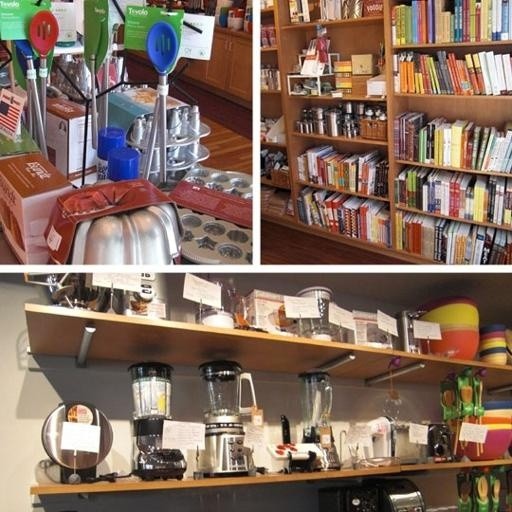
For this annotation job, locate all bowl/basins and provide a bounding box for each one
[195,309,234,328]
[479,324,508,366]
[412,297,479,363]
[447,401,511,458]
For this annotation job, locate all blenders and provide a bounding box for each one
[199,358,341,479]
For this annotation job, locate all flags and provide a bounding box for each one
[0,101,20,134]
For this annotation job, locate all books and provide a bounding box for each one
[260,1,512,265]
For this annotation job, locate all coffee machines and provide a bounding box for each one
[130,361,187,481]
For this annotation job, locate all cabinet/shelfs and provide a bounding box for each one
[178,30,251,102]
[260,0,511,264]
[23,308,511,494]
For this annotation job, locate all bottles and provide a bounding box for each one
[57,53,126,97]
[294,286,343,345]
[96,105,202,186]
[296,101,387,141]
